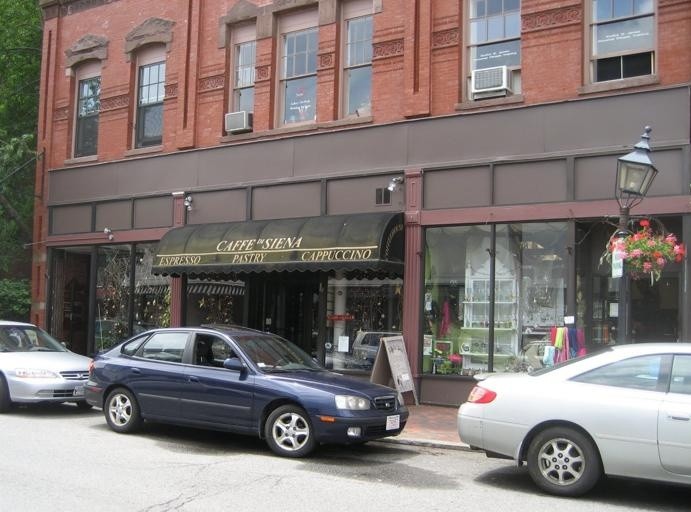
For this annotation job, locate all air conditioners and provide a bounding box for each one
[469,64,514,102]
[224,109,253,133]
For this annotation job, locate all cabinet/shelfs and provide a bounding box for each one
[519,250,563,333]
[459,235,522,377]
[585,273,610,352]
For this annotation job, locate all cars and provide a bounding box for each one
[65,320,148,353]
[84,324,410,458]
[0,319,96,410]
[326,352,374,370]
[456,341,691,499]
[514,342,547,372]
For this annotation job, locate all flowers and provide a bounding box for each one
[607,217,685,286]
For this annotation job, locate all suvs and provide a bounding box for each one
[351,331,403,368]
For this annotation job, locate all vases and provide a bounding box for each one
[625,269,652,280]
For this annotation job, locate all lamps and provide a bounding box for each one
[373,174,404,206]
[102,223,114,240]
[183,195,193,211]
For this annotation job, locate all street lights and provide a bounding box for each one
[614,124,659,343]
